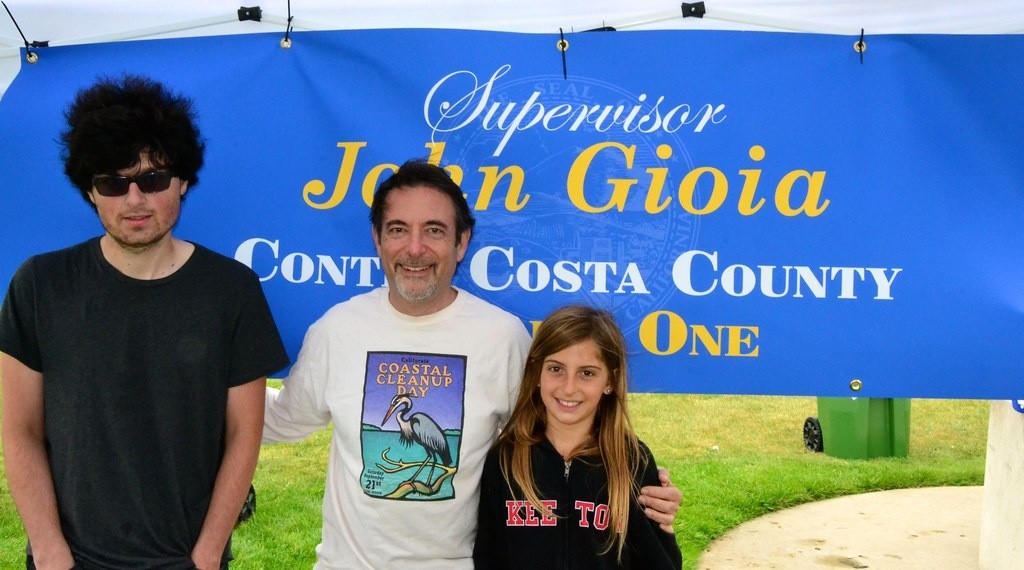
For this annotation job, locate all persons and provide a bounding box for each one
[261,158,682,570]
[473,307,683,570]
[0,69,292,570]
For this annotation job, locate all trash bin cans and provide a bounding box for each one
[803,396,913,461]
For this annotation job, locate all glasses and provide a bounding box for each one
[92,170,177,196]
